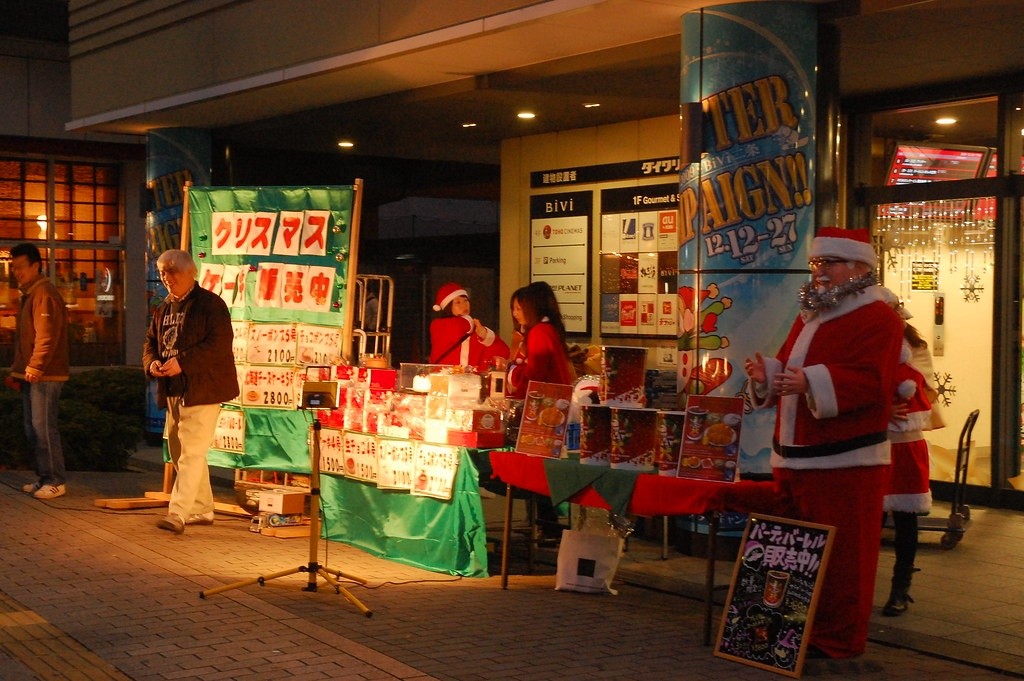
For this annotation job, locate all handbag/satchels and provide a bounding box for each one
[554,529,625,595]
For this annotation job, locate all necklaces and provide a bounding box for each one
[797,273,877,311]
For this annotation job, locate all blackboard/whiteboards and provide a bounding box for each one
[712,512,836,680]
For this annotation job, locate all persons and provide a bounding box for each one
[881,285,944,617]
[428,283,510,374]
[743,224,904,658]
[6,243,70,499]
[143,249,240,534]
[489,281,577,547]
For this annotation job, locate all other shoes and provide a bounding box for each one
[805,644,832,659]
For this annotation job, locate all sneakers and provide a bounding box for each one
[185,510,214,525]
[24,480,42,492]
[33,483,66,499]
[155,514,185,535]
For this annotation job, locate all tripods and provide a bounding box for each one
[199,418,373,620]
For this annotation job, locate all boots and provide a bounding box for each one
[882,564,921,616]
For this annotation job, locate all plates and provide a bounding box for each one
[540,408,565,427]
[704,424,736,446]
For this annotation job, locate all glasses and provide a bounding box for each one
[11,263,30,271]
[808,259,850,269]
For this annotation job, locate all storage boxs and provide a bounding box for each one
[258,491,305,514]
[329,364,510,446]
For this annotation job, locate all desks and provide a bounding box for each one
[309,427,513,577]
[486,452,780,646]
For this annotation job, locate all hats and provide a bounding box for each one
[432,281,469,311]
[809,226,877,270]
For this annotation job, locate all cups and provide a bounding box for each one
[687,406,709,439]
[525,391,544,420]
